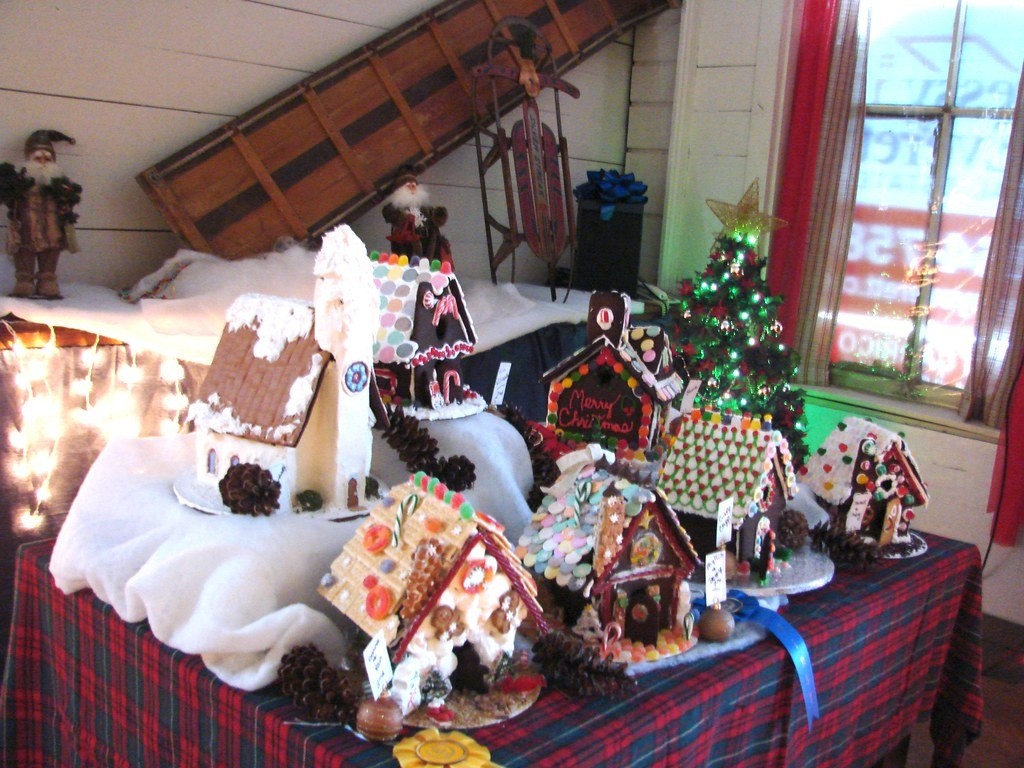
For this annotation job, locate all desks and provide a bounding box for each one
[0,527,983,768]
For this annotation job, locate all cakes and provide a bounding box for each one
[189,223,929,745]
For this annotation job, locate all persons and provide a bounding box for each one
[381,176,447,259]
[0,129,85,301]
